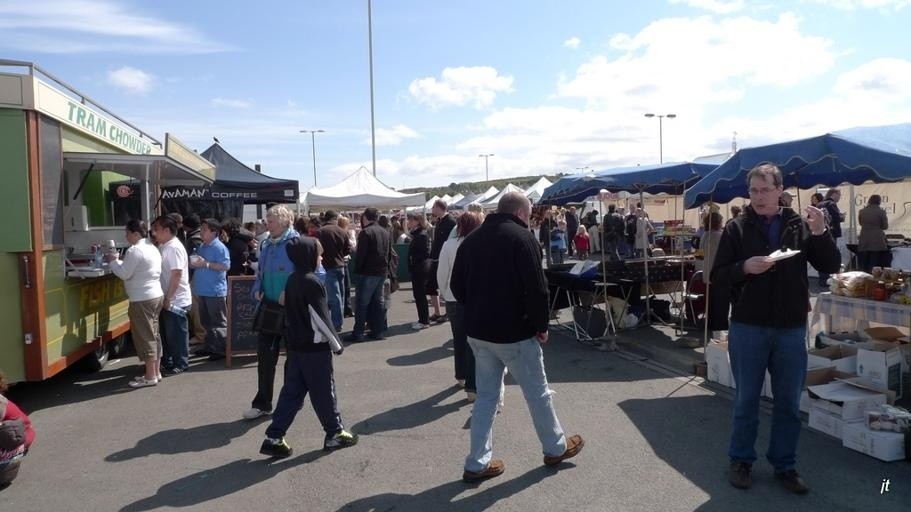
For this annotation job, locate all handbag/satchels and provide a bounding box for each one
[251,300,286,334]
[604,226,618,242]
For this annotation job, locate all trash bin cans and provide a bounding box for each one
[574,306,606,346]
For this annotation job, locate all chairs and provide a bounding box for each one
[680,270,706,334]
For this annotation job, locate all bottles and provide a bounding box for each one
[867,403,909,434]
[93,251,102,268]
[870,265,904,302]
[829,330,860,342]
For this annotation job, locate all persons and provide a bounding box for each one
[104,163,893,494]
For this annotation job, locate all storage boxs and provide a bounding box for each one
[706,327,911,462]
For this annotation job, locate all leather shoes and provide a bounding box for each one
[544,435,585,464]
[729,458,811,493]
[463,460,506,482]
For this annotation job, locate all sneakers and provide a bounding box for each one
[129,348,222,388]
[344,328,386,344]
[412,312,449,331]
[242,402,359,457]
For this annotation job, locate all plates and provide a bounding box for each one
[765,249,800,263]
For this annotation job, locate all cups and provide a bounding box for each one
[90,244,101,253]
[189,255,199,265]
[105,239,115,253]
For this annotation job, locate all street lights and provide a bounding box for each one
[300,129,325,187]
[479,153,496,181]
[645,113,675,166]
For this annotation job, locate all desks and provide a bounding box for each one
[811,291,910,342]
[545,269,634,343]
[624,253,696,326]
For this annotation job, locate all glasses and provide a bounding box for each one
[748,188,776,196]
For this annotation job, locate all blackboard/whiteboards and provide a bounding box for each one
[226,276,287,354]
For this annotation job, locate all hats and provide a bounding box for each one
[180,213,200,229]
